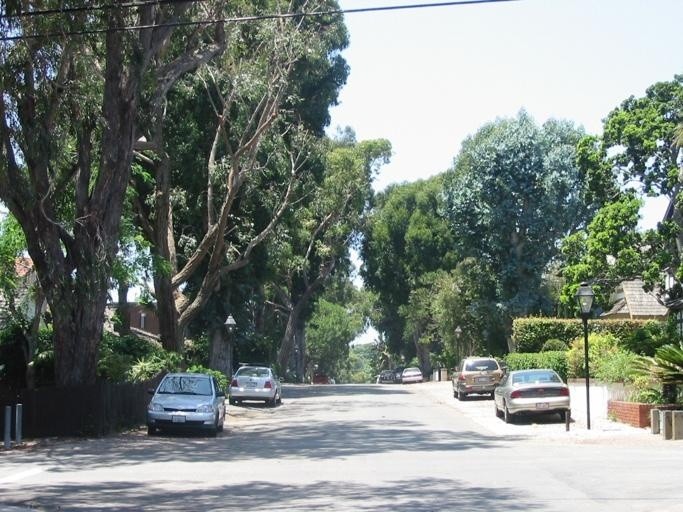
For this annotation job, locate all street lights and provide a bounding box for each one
[453,325,463,365]
[399,354,406,372]
[293,344,300,382]
[572,281,598,432]
[224,312,238,382]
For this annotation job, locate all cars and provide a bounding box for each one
[487,368,571,426]
[447,355,506,402]
[372,364,425,386]
[145,373,226,439]
[225,364,283,406]
